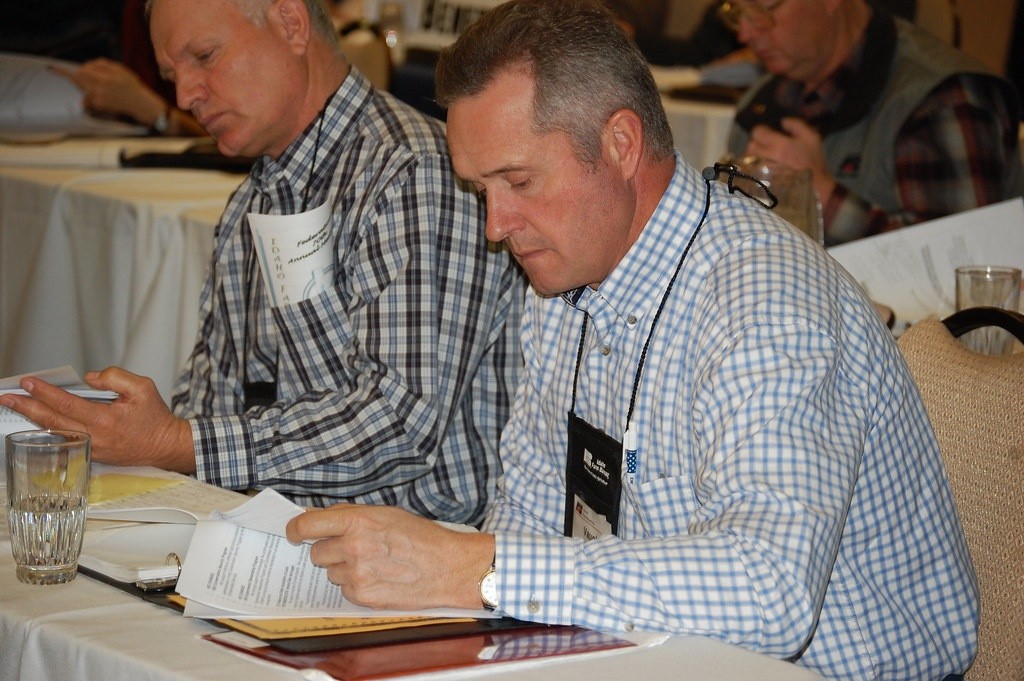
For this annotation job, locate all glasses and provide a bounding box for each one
[719,0,788,31]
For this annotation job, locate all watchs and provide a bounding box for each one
[153,104,173,133]
[477,551,498,611]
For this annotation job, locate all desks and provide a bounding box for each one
[654,60,740,172]
[0,434,824,681]
[0,138,251,418]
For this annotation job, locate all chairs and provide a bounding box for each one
[893,306,1024,681]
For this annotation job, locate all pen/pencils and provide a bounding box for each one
[621,420,637,488]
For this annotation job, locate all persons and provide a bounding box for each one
[0,0,530,533]
[45,0,387,137]
[616,0,1020,255]
[286,0,982,681]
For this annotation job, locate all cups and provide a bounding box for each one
[954,266,1022,356]
[716,153,823,249]
[5,430,91,586]
